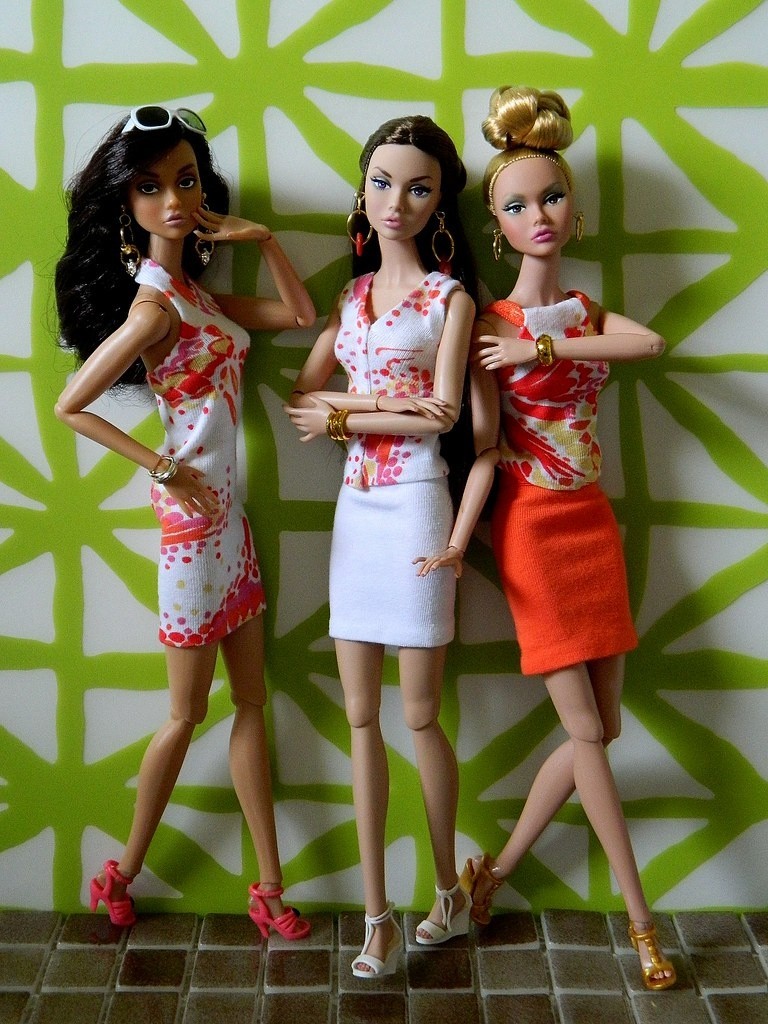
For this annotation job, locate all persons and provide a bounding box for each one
[56,108,315,943]
[415,85,678,987]
[282,116,477,976]
